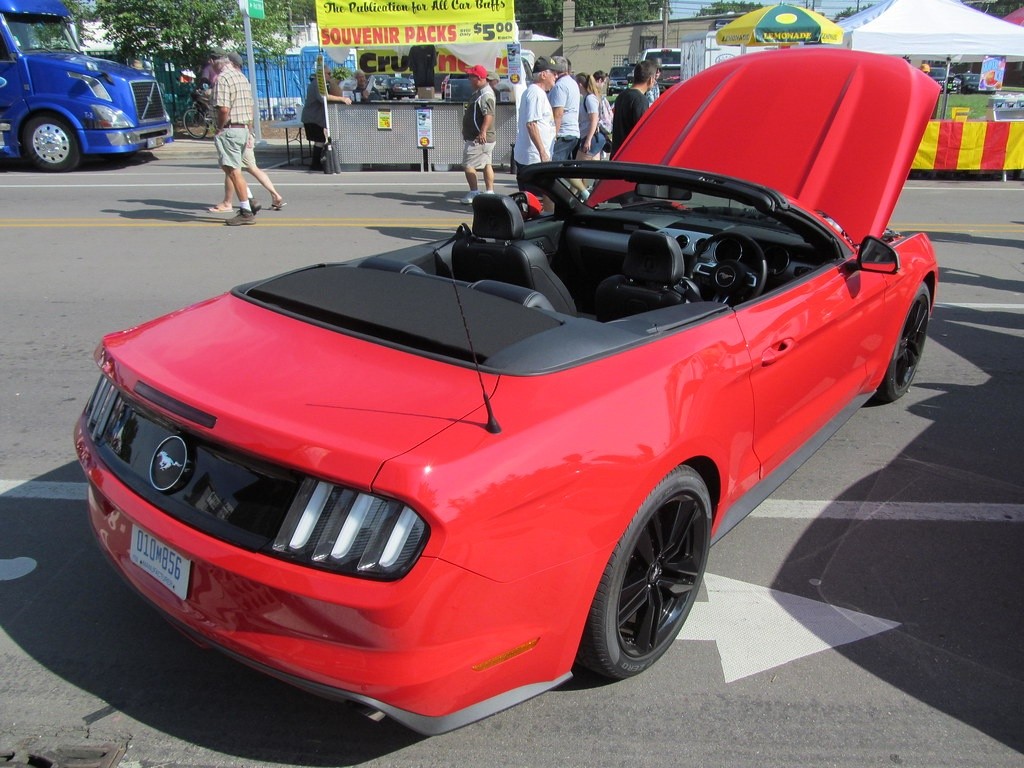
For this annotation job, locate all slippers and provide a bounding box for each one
[208,205,232,213]
[269,202,287,210]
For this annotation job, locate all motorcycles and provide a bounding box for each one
[183,83,215,139]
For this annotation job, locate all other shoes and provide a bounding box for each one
[460,193,477,204]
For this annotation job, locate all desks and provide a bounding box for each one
[911,119,1024,183]
[271,121,311,166]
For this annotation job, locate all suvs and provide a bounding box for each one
[607,66,637,95]
[639,48,681,94]
[929,66,954,94]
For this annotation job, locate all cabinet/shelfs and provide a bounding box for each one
[327,97,515,170]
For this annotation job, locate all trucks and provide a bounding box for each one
[681,20,803,83]
[73,49,931,737]
[521,48,536,72]
[0,0,174,172]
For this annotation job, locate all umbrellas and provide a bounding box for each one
[715,5,843,47]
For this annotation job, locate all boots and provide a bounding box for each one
[309,146,323,171]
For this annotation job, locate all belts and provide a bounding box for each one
[223,124,245,128]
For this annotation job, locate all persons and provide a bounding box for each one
[486,70,499,88]
[514,55,564,212]
[919,62,932,75]
[194,56,220,122]
[207,46,287,227]
[609,61,659,162]
[563,72,606,205]
[301,64,352,171]
[645,56,663,108]
[353,70,383,101]
[547,56,600,209]
[460,65,497,205]
[593,70,608,97]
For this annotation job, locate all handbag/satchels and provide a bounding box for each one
[579,132,606,156]
[603,132,613,152]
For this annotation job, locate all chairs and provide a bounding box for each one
[451,195,594,326]
[952,107,970,119]
[594,231,706,324]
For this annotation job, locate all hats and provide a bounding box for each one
[486,73,500,82]
[229,52,243,69]
[532,56,564,73]
[464,65,487,79]
[208,47,227,58]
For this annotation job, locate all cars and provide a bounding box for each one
[381,77,417,100]
[367,74,392,96]
[956,74,996,94]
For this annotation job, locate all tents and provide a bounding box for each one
[791,0,1024,120]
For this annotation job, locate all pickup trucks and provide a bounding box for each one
[440,57,588,103]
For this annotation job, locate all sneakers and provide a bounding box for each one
[225,208,256,225]
[249,197,261,215]
